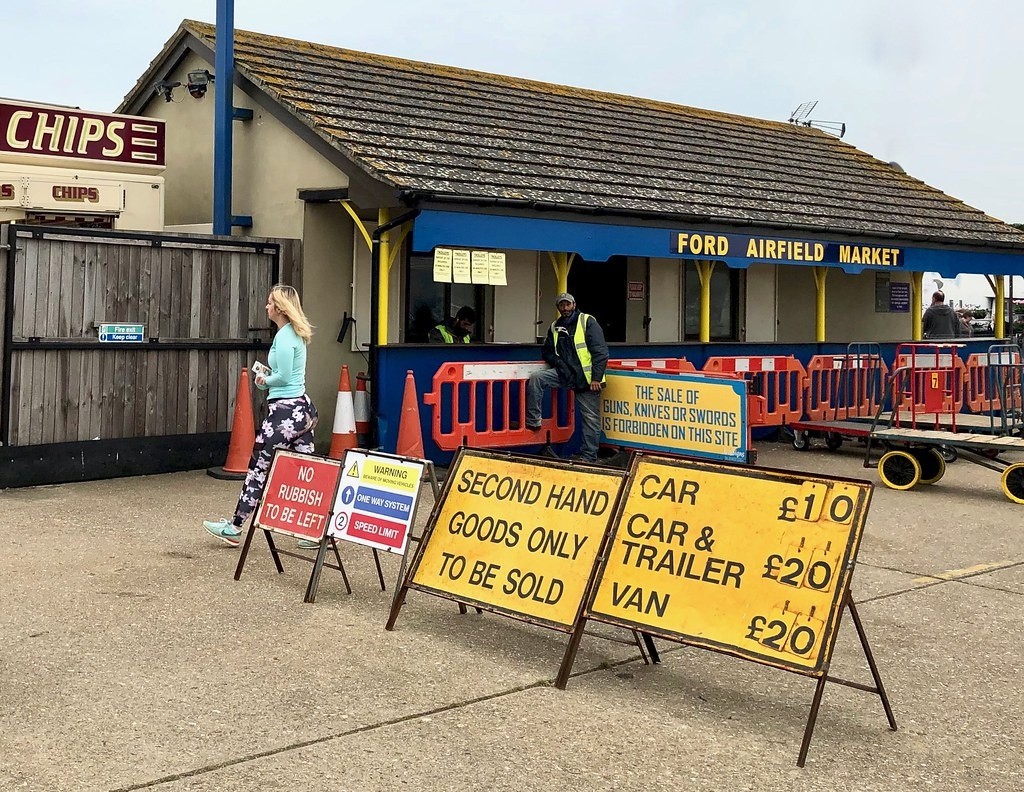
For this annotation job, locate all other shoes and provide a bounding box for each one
[511,418,541,431]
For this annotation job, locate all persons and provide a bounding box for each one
[428,305,476,344]
[958,313,974,337]
[202,285,340,549]
[922,291,960,339]
[511,292,609,462]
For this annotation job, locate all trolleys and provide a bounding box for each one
[789,341,1024,504]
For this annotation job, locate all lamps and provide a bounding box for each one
[187,69,215,86]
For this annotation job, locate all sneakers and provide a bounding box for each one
[298,534,340,549]
[203,517,243,546]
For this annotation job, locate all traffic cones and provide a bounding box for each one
[207,368,255,480]
[354,372,372,449]
[329,365,359,461]
[397,370,449,482]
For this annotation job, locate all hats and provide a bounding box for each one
[555,294,574,305]
